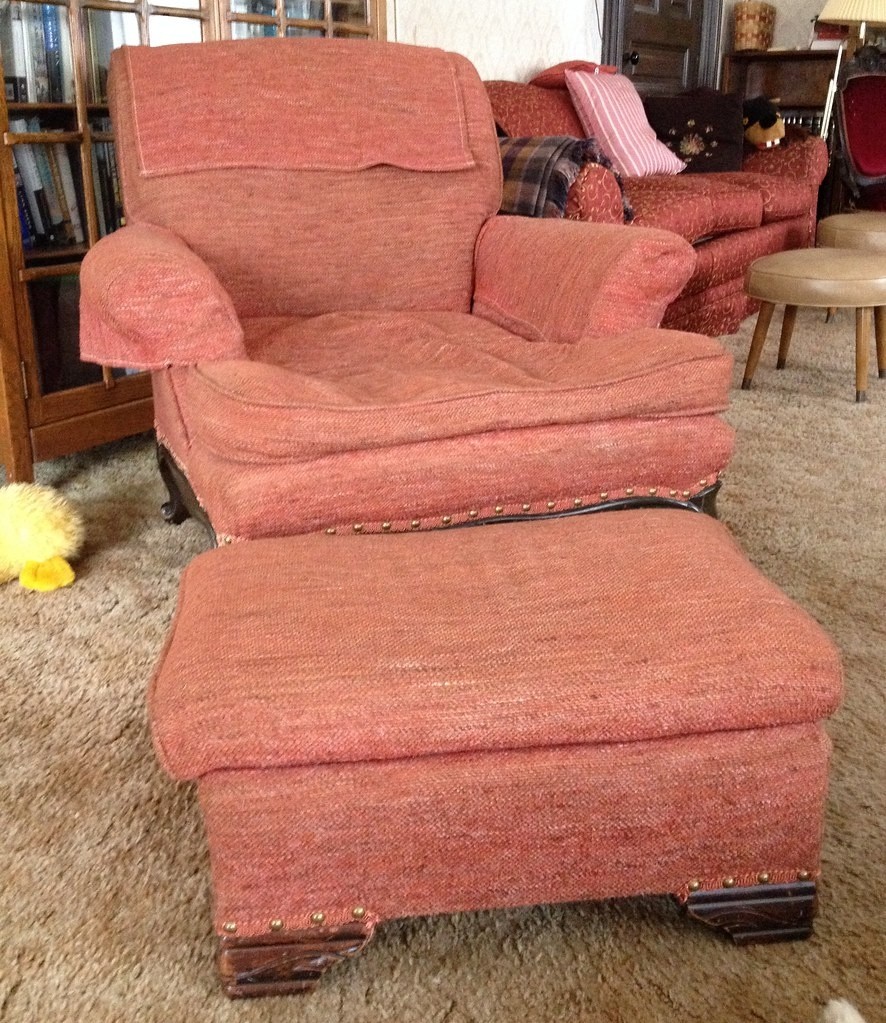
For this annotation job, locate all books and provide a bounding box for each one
[0,1,108,104]
[783,116,840,168]
[8,114,126,249]
[233,0,324,39]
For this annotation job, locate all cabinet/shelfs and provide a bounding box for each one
[0,0,388,491]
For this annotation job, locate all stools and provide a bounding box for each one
[146,505,848,1007]
[741,245,886,402]
[815,208,886,324]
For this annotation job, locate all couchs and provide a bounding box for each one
[75,42,733,547]
[473,79,828,341]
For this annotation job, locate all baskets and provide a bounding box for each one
[733,1,776,54]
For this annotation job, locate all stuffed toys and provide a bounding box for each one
[744,96,785,148]
[0,482,86,591]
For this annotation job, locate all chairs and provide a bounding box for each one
[836,57,885,211]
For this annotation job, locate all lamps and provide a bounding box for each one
[817,0,886,54]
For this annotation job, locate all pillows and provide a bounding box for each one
[530,62,624,95]
[641,89,748,174]
[563,69,690,180]
